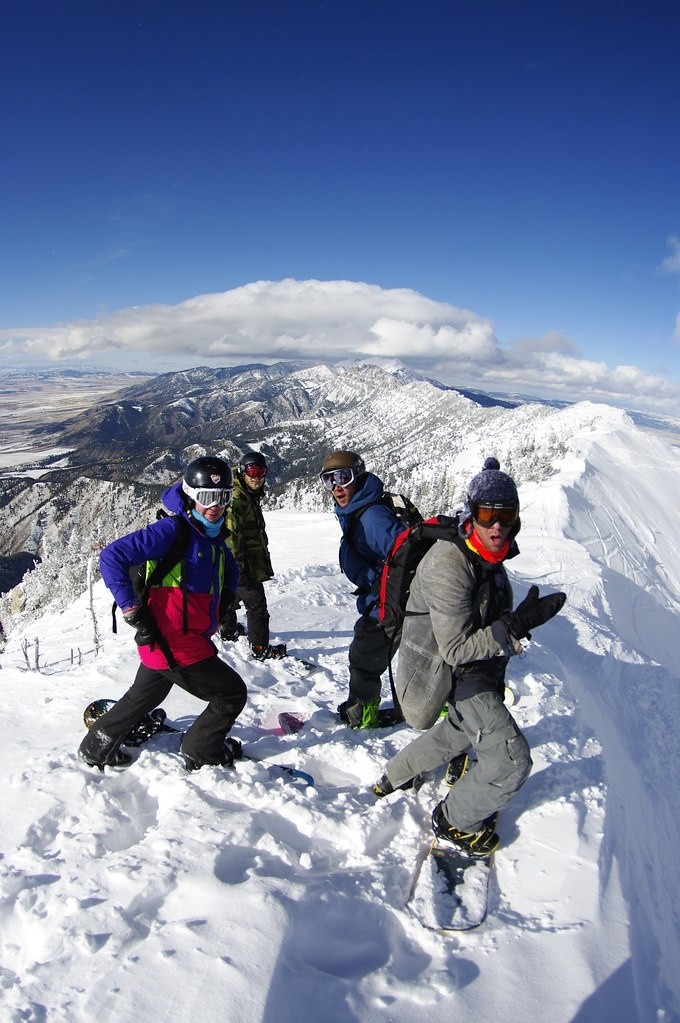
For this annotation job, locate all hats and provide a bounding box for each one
[458,457,521,546]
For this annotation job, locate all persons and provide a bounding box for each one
[77,456,247,773]
[320,450,449,730]
[372,456,567,855]
[218,452,287,662]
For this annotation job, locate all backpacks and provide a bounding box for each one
[122,515,230,604]
[345,491,424,550]
[378,517,486,647]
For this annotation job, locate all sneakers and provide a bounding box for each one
[432,801,500,855]
[185,737,243,773]
[372,769,416,797]
[87,748,132,769]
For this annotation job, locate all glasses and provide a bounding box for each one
[471,502,519,528]
[193,488,233,509]
[237,452,268,475]
[244,465,266,478]
[320,468,354,492]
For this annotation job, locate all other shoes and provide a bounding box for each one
[221,623,244,641]
[337,700,376,725]
[251,644,285,661]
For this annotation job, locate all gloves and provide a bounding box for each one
[126,605,158,646]
[236,558,249,589]
[502,585,567,642]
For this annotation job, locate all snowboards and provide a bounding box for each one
[237,633,318,680]
[83,696,316,790]
[404,751,499,935]
[278,684,516,737]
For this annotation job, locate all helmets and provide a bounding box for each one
[319,451,365,491]
[183,456,232,510]
